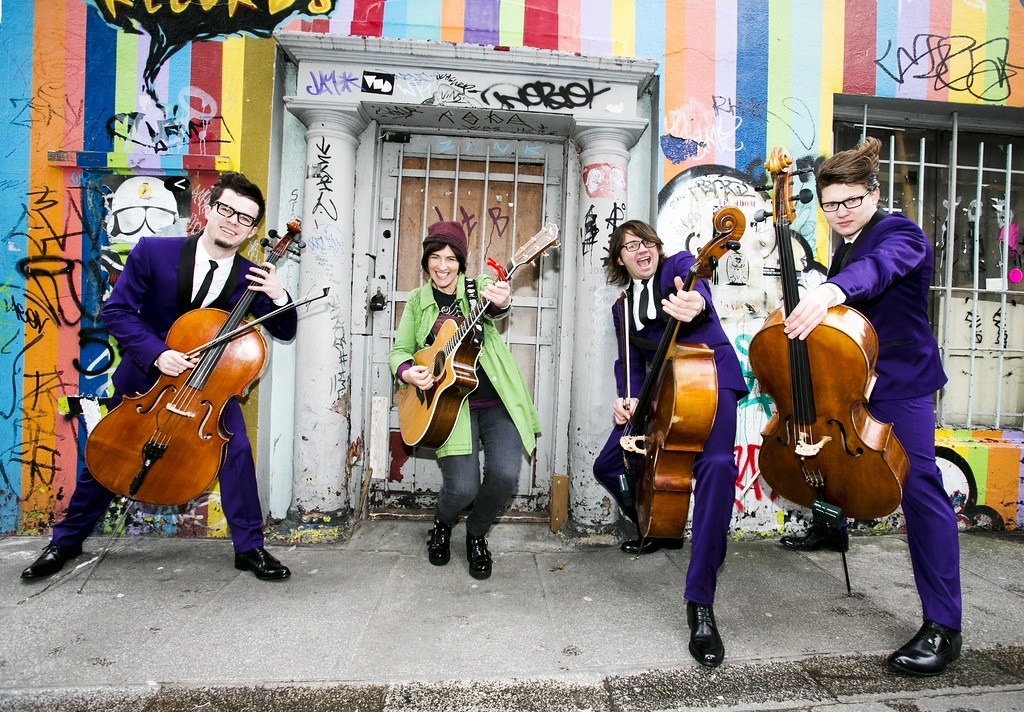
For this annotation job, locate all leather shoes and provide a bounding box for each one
[429,522,453,566]
[686,601,725,666]
[468,534,494,580]
[18,544,83,579]
[233,546,292,582]
[889,623,963,677]
[620,536,684,555]
[779,527,851,551]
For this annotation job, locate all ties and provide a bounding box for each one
[827,242,852,280]
[639,279,653,325]
[192,259,219,307]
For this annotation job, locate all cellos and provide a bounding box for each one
[78,219,306,593]
[748,145,910,598]
[618,206,746,561]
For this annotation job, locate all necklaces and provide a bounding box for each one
[436,285,457,295]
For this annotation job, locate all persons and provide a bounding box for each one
[779,136,963,676]
[390,221,541,579]
[20,172,299,579]
[593,221,749,667]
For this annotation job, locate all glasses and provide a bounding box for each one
[819,188,873,212]
[212,200,258,227]
[619,239,656,251]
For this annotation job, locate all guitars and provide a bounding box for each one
[398,222,562,451]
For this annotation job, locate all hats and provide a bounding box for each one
[424,221,468,258]
